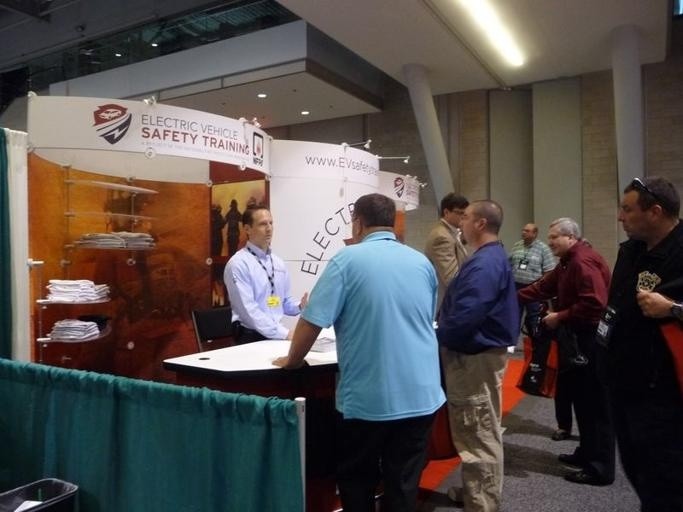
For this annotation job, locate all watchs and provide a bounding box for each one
[670,301,683,320]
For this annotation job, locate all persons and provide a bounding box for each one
[224,203,308,344]
[607,174,683,512]
[508,222,556,326]
[424,193,468,313]
[515,216,615,486]
[551,238,593,440]
[273,193,447,512]
[435,200,520,512]
[224,199,243,256]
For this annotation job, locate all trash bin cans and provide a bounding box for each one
[0,477,79,512]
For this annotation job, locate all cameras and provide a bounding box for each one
[518,301,554,393]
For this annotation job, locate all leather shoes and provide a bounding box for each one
[558,454,581,466]
[565,470,615,486]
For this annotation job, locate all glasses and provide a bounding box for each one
[629,177,658,200]
[452,210,464,215]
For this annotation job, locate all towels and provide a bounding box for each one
[47,318,100,341]
[79,231,157,251]
[44,279,111,302]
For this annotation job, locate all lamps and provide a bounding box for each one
[420,182,428,189]
[239,116,262,130]
[143,96,156,106]
[347,139,371,149]
[377,156,410,164]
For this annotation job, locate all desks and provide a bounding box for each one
[162,319,448,512]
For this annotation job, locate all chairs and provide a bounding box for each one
[190,306,239,353]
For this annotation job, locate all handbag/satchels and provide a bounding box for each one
[515,336,558,398]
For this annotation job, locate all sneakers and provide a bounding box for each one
[551,429,569,441]
[447,486,464,502]
